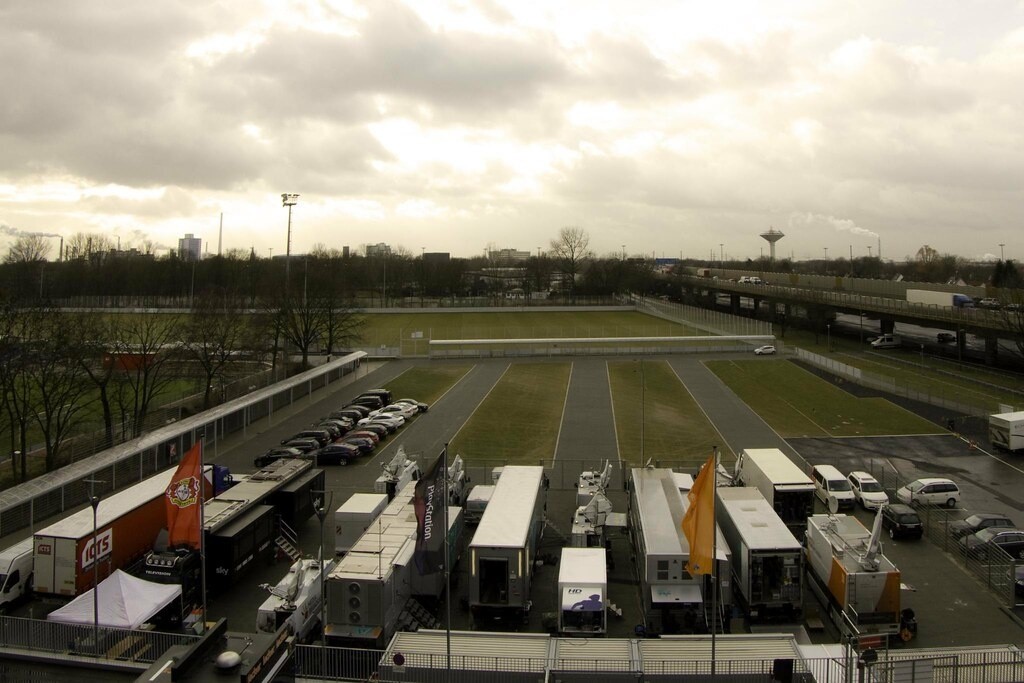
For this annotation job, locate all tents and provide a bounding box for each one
[47,569,183,635]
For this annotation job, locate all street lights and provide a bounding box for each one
[282,192,300,256]
[311,488,333,683]
[90,494,103,656]
[826,324,832,354]
[621,244,628,259]
[867,245,872,257]
[824,247,828,261]
[998,242,1005,268]
[719,243,725,266]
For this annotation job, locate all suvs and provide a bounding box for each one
[948,513,1016,540]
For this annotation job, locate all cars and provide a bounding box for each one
[253,389,429,468]
[1003,303,1024,314]
[959,525,1024,561]
[847,471,890,511]
[1006,563,1024,596]
[873,504,924,542]
[755,345,776,356]
[729,279,735,283]
[937,333,956,342]
[896,478,960,508]
[980,298,1003,309]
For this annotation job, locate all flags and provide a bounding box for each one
[414,451,446,576]
[682,455,714,574]
[165,442,200,550]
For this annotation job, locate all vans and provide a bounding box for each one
[811,465,855,510]
[738,276,762,284]
[871,334,902,349]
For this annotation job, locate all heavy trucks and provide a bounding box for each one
[469,448,917,647]
[144,456,324,619]
[33,462,214,608]
[906,289,975,309]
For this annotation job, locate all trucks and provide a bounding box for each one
[374,461,421,498]
[0,535,33,615]
